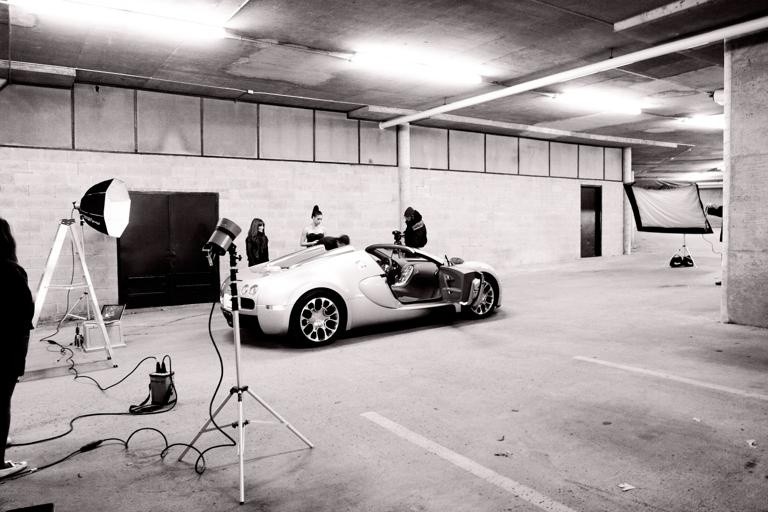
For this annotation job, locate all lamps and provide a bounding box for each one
[176,218,315,507]
[25,178,130,372]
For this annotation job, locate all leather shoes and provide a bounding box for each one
[0,460,28,479]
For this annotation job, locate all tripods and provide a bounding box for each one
[175,260,314,506]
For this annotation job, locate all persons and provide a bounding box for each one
[299,204,324,247]
[403,206,428,248]
[245,218,269,267]
[0,218,37,479]
[707,203,723,285]
[316,234,350,250]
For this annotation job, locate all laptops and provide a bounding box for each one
[85,302,126,326]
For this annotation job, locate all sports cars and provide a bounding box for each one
[220,245,501,349]
